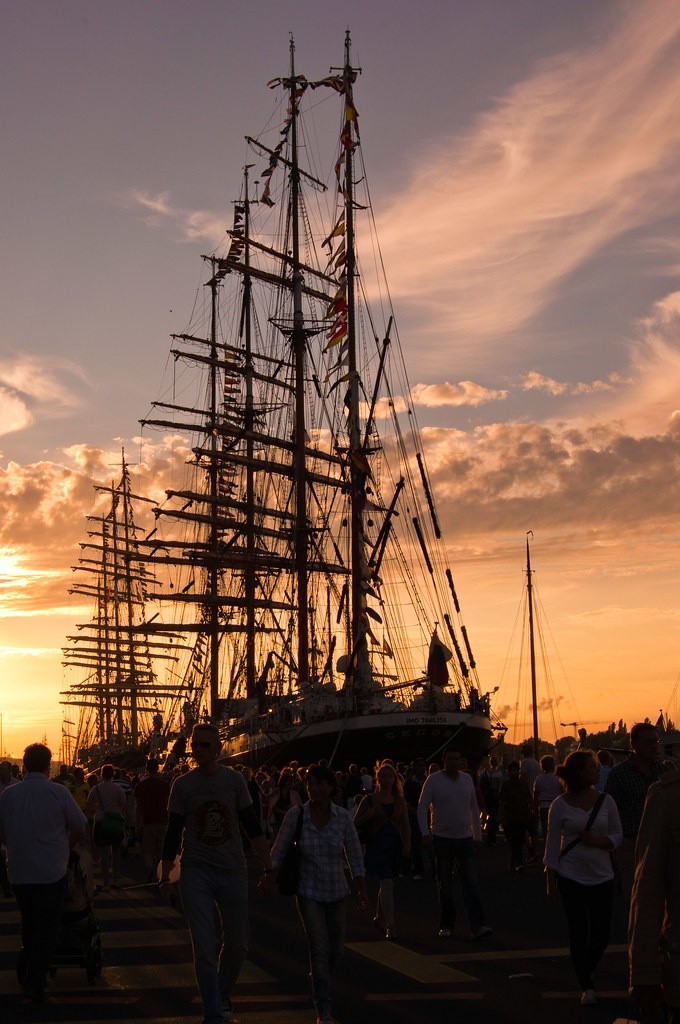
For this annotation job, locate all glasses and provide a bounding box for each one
[191,742,210,748]
[382,774,394,778]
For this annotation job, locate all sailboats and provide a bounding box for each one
[60,26,506,770]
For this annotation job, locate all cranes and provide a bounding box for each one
[561,722,598,741]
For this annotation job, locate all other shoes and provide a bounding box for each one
[438,930,452,937]
[220,998,231,1018]
[24,976,44,999]
[581,989,598,1006]
[373,918,394,943]
[319,1015,335,1024]
[16,954,31,985]
[471,927,493,937]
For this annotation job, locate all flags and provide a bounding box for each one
[93,62,454,746]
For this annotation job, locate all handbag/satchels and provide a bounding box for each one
[354,794,381,844]
[96,812,126,848]
[613,1004,647,1024]
[277,806,306,897]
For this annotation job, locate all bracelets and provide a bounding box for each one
[264,869,274,875]
[360,896,369,901]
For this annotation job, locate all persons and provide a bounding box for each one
[0,723,680,1024]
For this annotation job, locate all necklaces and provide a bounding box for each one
[311,805,328,821]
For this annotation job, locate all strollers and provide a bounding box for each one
[17,848,103,989]
